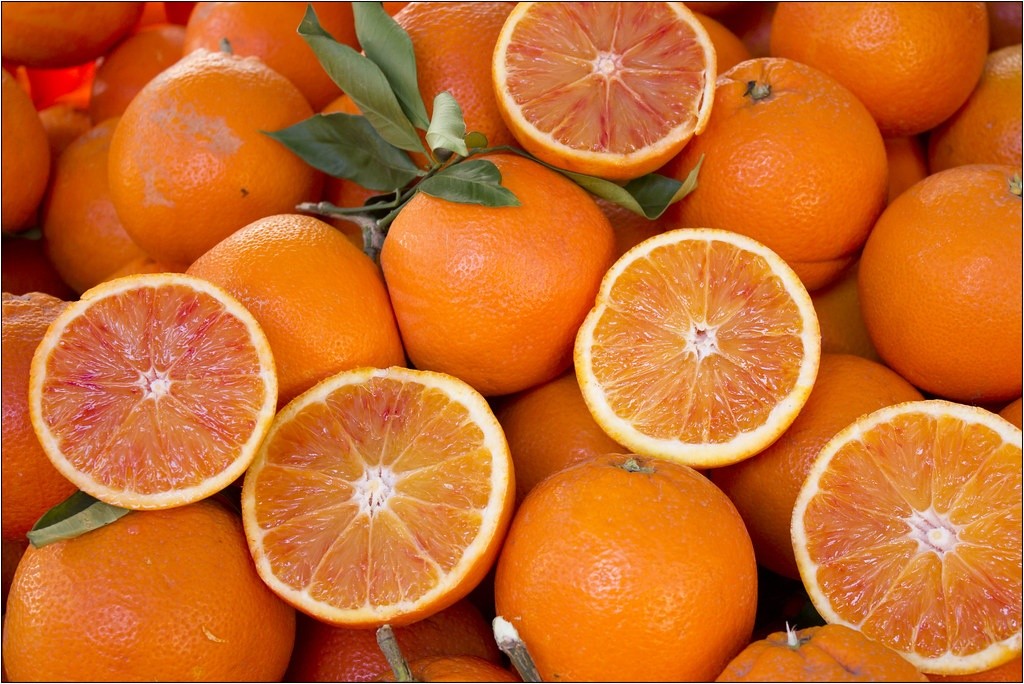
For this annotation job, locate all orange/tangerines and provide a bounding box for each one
[2,2,1022,682]
